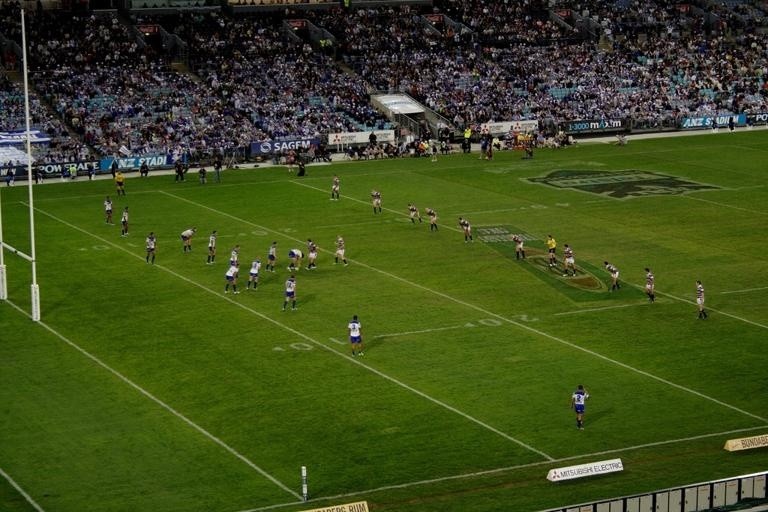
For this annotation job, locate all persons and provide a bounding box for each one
[512,235,526,260]
[695,281,708,318]
[604,261,620,290]
[0,0,767,194]
[572,385,589,430]
[425,208,438,231]
[225,239,318,313]
[104,197,130,235]
[329,173,340,201]
[348,315,364,357]
[644,267,656,303]
[333,235,348,266]
[145,232,156,265]
[181,228,198,253]
[545,235,576,276]
[408,204,422,224]
[370,189,383,214]
[458,217,473,243]
[206,231,217,265]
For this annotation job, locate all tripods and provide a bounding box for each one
[227,151,242,168]
[222,152,235,169]
